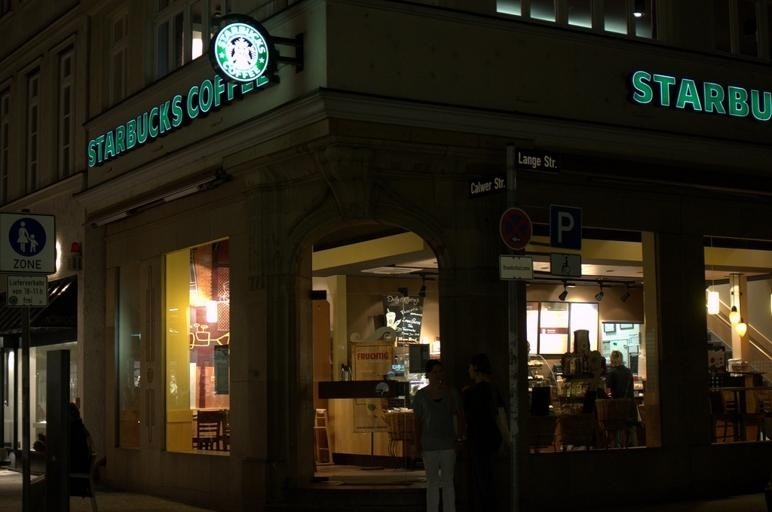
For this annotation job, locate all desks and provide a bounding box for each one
[723,369,767,442]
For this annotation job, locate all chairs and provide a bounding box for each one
[70,456,106,512]
[529,387,772,453]
[220,410,231,451]
[192,410,224,450]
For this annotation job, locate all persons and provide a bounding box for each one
[413,358,466,512]
[462,352,510,512]
[604,350,632,449]
[30,401,96,511]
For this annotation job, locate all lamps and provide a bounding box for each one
[708,280,722,317]
[592,281,604,303]
[419,277,427,300]
[618,282,632,304]
[728,277,747,338]
[555,282,570,303]
